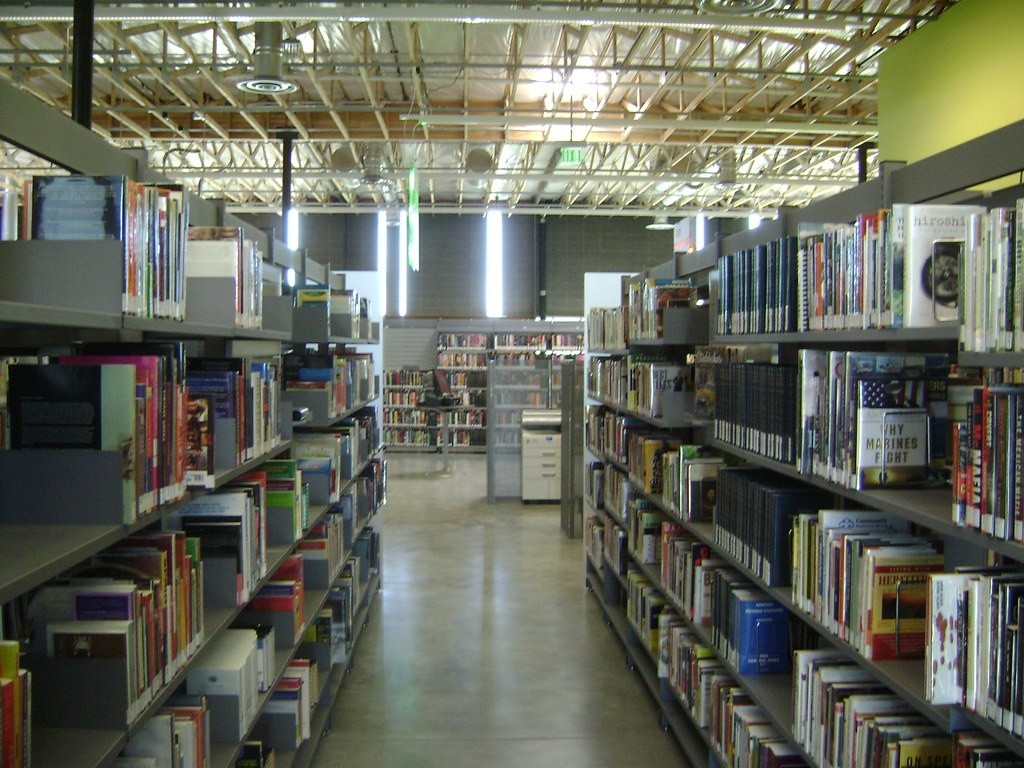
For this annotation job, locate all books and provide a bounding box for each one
[0,174,1022,768]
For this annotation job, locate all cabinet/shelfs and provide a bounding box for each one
[0,75,387,768]
[586,120,1024,768]
[382,332,586,505]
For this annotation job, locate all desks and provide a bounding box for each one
[413,405,475,479]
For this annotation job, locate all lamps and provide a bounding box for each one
[235,1,297,94]
[359,145,384,185]
[714,152,742,191]
[693,0,779,13]
[644,217,675,230]
